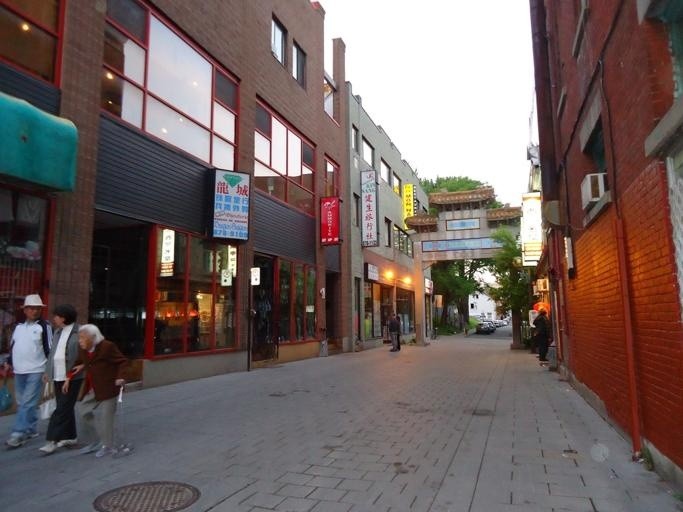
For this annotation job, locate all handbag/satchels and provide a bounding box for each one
[39,397,57,419]
[0,386,13,412]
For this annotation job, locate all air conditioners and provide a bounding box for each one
[580,172,610,211]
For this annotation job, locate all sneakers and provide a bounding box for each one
[5,431,113,458]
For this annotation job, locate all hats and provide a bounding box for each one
[20,294,47,309]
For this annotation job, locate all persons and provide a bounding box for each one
[37,303,87,454]
[252,287,271,353]
[3,294,53,447]
[533,310,552,360]
[61,324,128,457]
[388,313,400,352]
[397,316,400,351]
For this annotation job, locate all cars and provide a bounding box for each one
[476,313,512,334]
